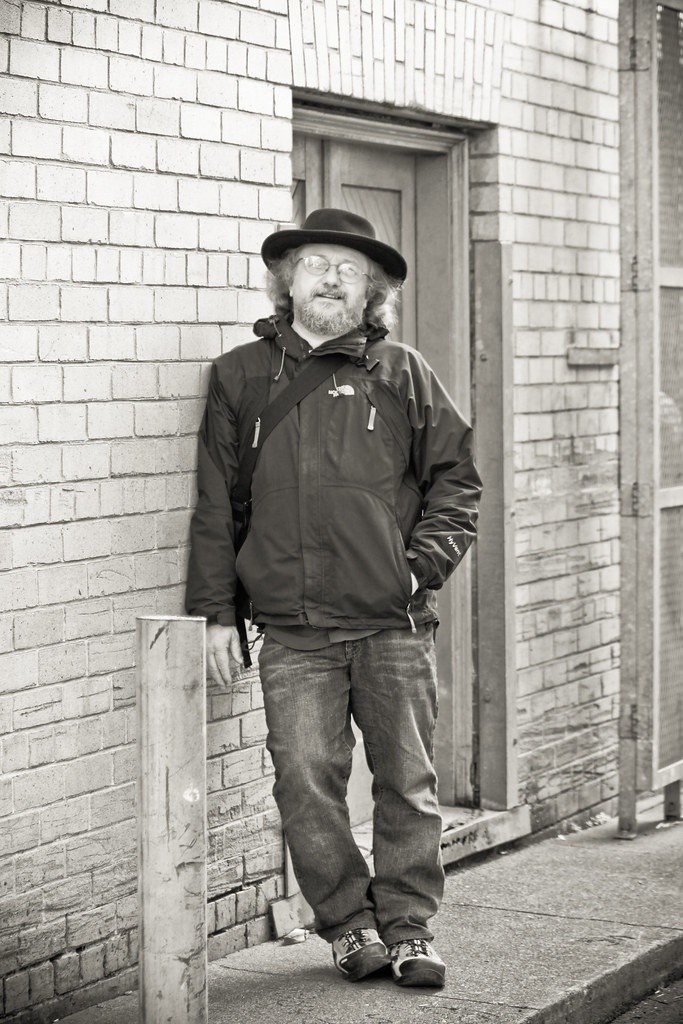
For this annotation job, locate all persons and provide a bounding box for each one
[184,210,482,990]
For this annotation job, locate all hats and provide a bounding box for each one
[261,210,407,286]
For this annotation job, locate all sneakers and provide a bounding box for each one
[389,939,445,987]
[332,928,390,983]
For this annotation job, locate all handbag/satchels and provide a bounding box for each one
[225,485,267,629]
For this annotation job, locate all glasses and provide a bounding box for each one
[292,255,372,285]
